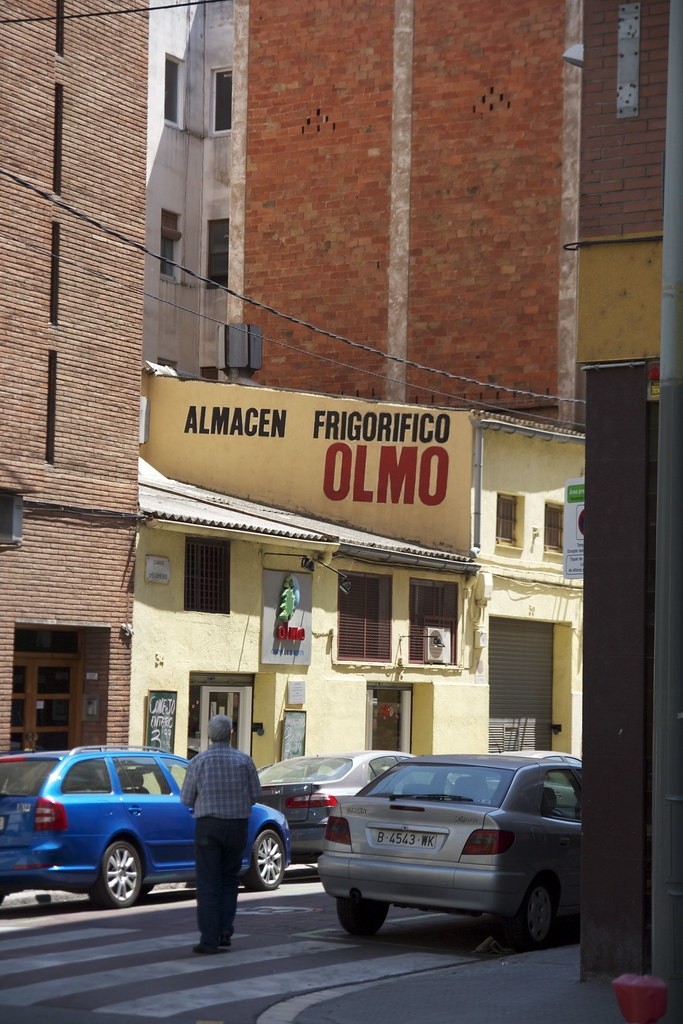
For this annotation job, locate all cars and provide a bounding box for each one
[315,756,583,954]
[251,750,453,857]
[495,750,584,806]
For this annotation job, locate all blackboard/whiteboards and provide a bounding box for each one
[144,689,178,755]
[280,708,307,761]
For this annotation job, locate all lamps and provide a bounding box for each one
[262,550,313,570]
[563,41,584,71]
[315,554,360,593]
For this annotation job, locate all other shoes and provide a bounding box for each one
[193,935,231,954]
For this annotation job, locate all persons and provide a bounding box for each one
[180,714,261,955]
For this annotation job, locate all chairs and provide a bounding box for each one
[452,775,489,803]
[541,788,557,815]
[118,769,148,794]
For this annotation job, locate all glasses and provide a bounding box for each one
[230,728,233,733]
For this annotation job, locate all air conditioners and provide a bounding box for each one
[423,628,450,664]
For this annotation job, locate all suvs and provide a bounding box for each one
[0,741,292,908]
